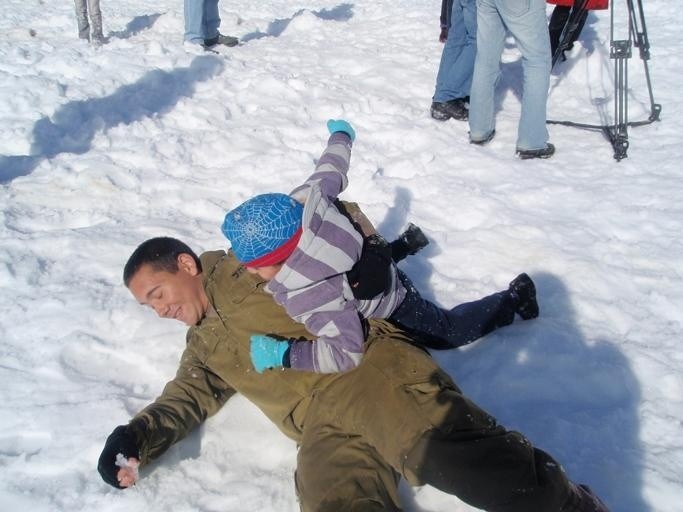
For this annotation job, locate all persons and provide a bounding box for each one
[96,201,608,512]
[183,0,239,56]
[75,0,105,50]
[222,119,539,373]
[430,0,608,158]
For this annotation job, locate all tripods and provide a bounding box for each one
[546,1,662,163]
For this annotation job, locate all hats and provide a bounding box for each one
[218,192,303,270]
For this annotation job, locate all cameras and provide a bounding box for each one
[388,223,430,264]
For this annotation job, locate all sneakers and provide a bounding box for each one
[430,94,469,120]
[508,271,539,322]
[204,32,240,47]
[514,139,556,160]
[467,129,496,145]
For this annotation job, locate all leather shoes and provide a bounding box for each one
[400,221,429,256]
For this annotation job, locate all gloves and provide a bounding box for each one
[248,331,288,374]
[94,423,143,490]
[323,118,360,140]
